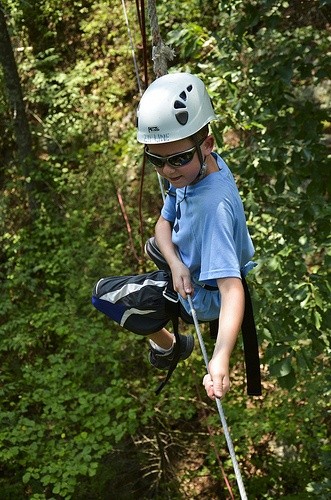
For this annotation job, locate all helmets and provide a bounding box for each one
[136,73,218,144]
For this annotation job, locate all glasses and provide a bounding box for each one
[144,136,207,168]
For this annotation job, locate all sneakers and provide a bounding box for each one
[150,334,194,370]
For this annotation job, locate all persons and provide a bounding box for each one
[89,72,257,401]
